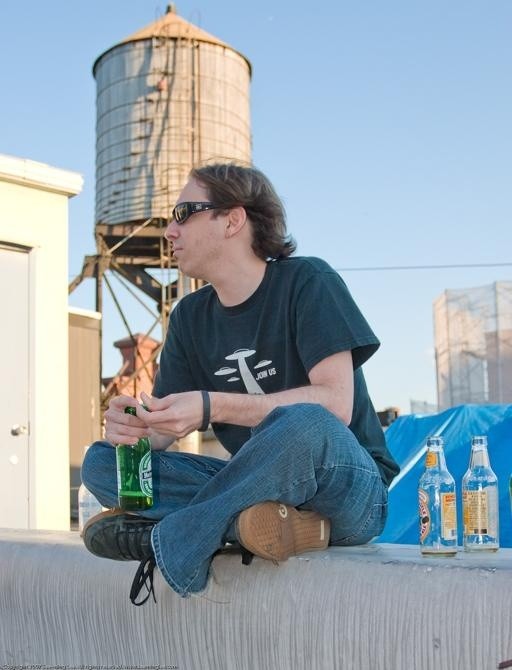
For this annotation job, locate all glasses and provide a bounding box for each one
[172,201,227,226]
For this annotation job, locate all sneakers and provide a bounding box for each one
[233,501,332,563]
[80,506,161,561]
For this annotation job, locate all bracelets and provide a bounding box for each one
[197,388,212,433]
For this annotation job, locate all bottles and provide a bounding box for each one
[460,435,500,551]
[78,483,103,537]
[417,437,458,555]
[115,405,155,511]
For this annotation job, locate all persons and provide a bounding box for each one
[77,158,400,599]
